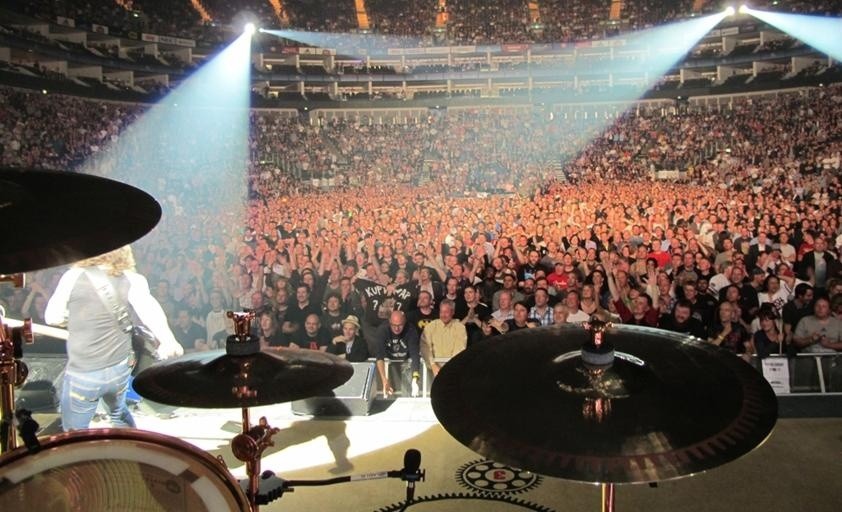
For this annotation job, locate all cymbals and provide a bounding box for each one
[431,324,780,486]
[0,167,161,274]
[132,346,354,408]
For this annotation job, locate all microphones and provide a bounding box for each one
[403,448,422,500]
[231,426,273,462]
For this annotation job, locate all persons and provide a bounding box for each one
[41,237,188,433]
[1,1,842,395]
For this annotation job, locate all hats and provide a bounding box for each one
[340,314,361,330]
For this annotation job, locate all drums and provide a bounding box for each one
[1,429,251,512]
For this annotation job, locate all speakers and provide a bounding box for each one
[290,361,378,417]
[15,352,68,410]
